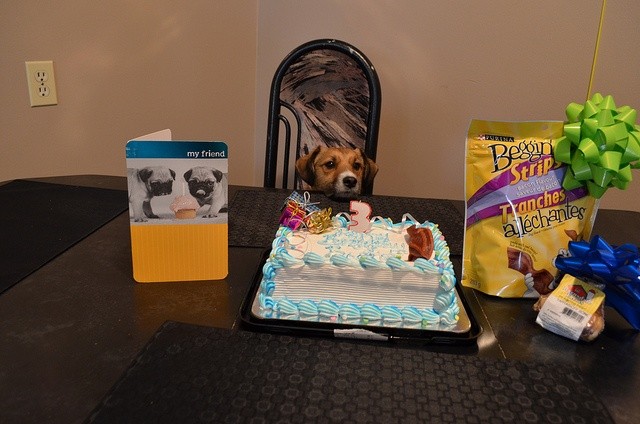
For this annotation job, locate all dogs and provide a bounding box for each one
[127,166,176,221]
[295,145,378,199]
[183,167,229,218]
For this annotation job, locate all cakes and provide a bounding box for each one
[258,190,460,331]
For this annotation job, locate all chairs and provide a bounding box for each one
[263,37,382,197]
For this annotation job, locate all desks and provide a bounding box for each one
[0,174,639,422]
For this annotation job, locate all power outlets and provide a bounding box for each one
[23,59,59,108]
